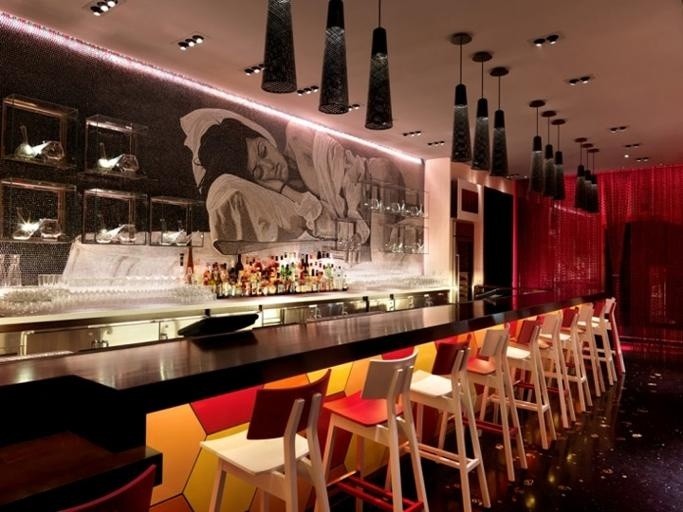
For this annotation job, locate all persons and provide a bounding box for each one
[193,116,423,266]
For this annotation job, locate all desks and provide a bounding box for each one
[0,291,610,512]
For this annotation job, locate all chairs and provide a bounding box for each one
[476,315,556,449]
[565,300,617,385]
[66,463,156,508]
[313,350,429,512]
[547,302,604,396]
[198,368,330,512]
[581,297,625,377]
[434,323,527,482]
[383,333,490,512]
[527,305,592,412]
[510,309,576,428]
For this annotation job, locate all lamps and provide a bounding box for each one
[540,110,557,197]
[528,99,545,194]
[574,137,587,208]
[489,66,509,177]
[363,1,392,130]
[470,51,493,172]
[550,118,565,200]
[582,143,593,210]
[587,148,599,213]
[449,33,472,162]
[261,0,296,93]
[318,0,347,115]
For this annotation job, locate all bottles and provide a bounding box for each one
[177,253,185,286]
[203,251,348,298]
[186,246,194,285]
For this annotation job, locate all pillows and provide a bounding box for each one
[205,174,306,243]
